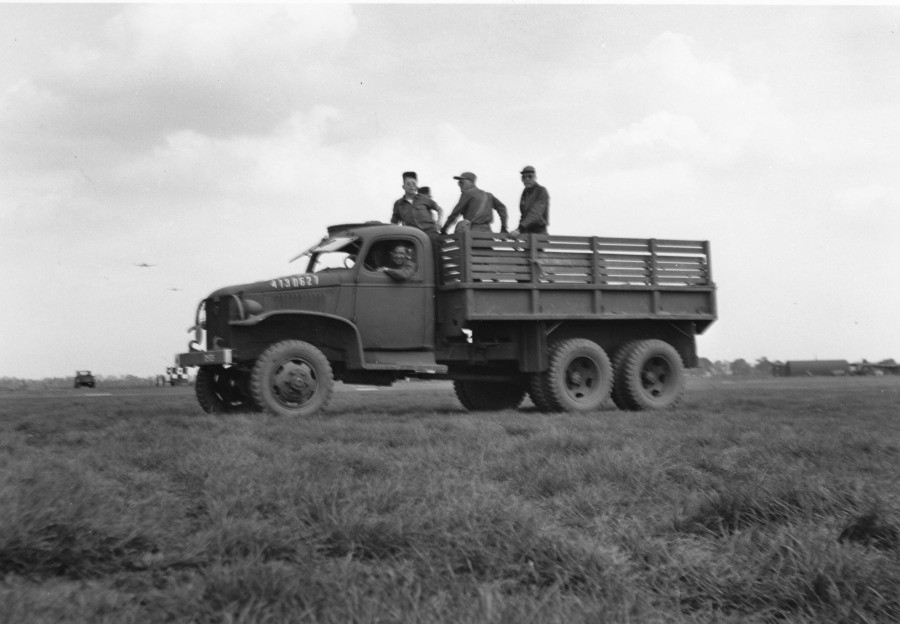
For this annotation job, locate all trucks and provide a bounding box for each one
[171,221,715,419]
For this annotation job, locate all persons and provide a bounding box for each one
[441,172,509,283]
[379,245,417,282]
[390,171,443,237]
[416,186,432,199]
[508,166,550,283]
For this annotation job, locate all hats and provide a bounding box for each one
[520,166,536,174]
[452,172,477,182]
